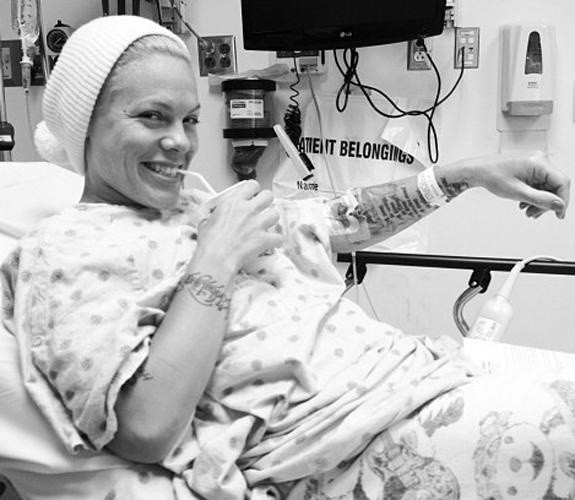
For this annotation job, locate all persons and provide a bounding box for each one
[15,15,575,499]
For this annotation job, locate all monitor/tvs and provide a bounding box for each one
[241,0,446,51]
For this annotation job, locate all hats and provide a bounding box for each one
[37,13,190,181]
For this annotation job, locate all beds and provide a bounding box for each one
[0,160,575,500]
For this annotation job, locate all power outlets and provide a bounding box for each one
[0,39,23,87]
[454,26,479,69]
[198,35,237,76]
[294,56,328,74]
[407,37,432,70]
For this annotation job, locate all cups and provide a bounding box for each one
[199,179,278,276]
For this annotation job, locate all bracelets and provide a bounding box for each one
[417,165,449,211]
